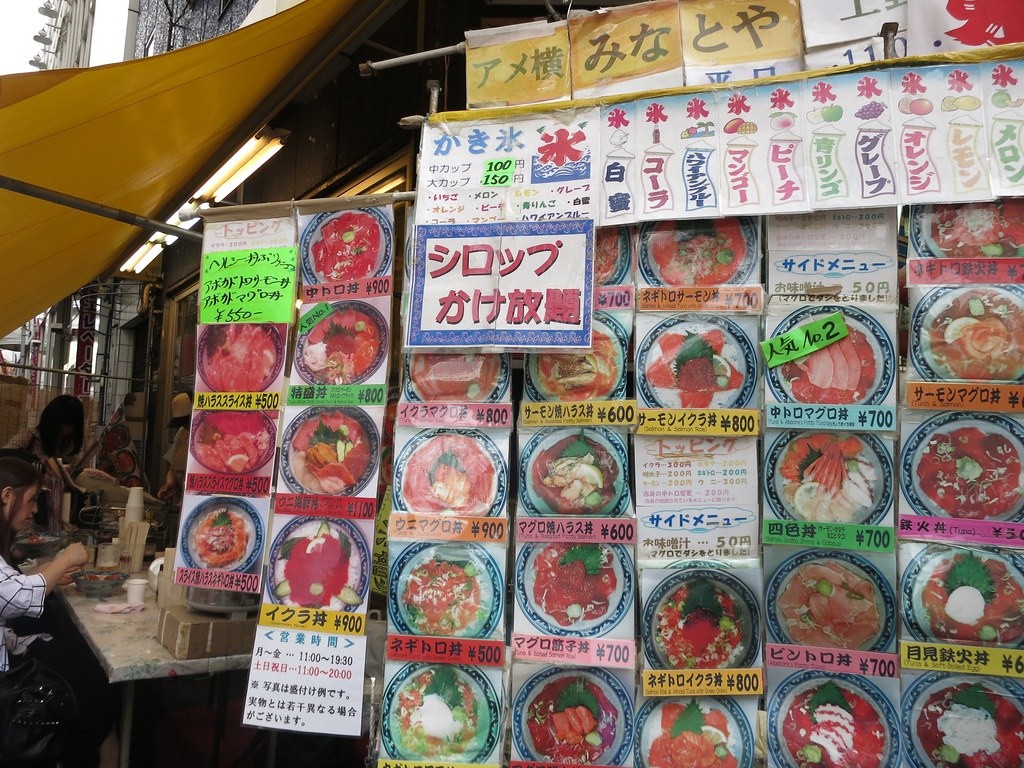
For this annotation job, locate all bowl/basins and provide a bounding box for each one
[71,570,130,598]
[96,401,138,484]
[13,531,67,557]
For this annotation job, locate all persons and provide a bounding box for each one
[3,392,86,467]
[156,390,193,502]
[0,455,124,768]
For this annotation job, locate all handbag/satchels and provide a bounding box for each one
[1,656,76,768]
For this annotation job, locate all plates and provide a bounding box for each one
[182,205,1024,768]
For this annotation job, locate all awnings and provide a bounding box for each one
[0,1,349,348]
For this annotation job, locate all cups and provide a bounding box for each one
[125,578,149,605]
[125,487,145,527]
[96,543,121,569]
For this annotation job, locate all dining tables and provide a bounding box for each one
[37,539,257,768]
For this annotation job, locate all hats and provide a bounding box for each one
[166,391,194,428]
